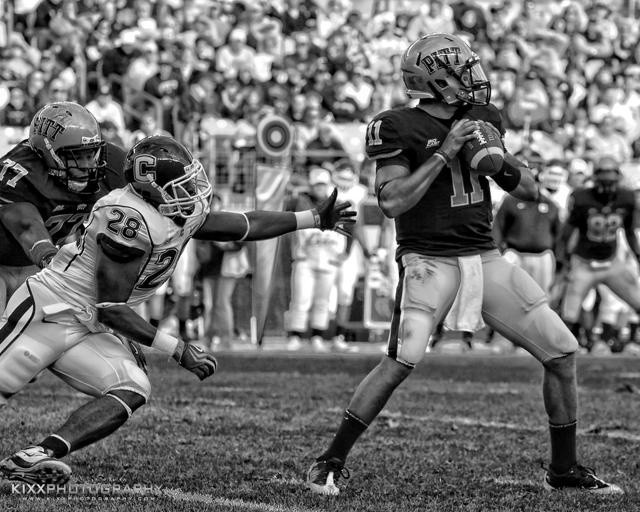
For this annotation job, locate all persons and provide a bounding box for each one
[305,28,626,497]
[0,135,357,495]
[0,0,640,355]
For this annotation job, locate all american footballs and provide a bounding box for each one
[461,121,504,174]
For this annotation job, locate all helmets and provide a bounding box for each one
[122,136,213,218]
[29,102,108,195]
[402,33,491,107]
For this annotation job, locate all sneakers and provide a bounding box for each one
[541,462,624,494]
[0,445,72,488]
[305,455,349,496]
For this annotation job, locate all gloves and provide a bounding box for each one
[175,341,216,379]
[314,187,357,237]
